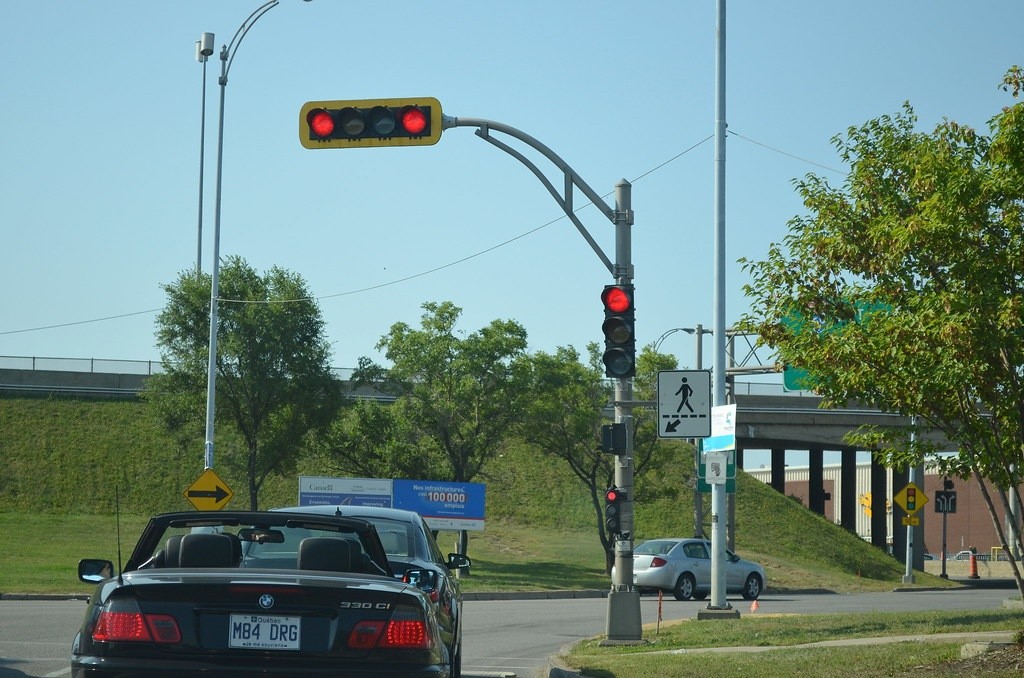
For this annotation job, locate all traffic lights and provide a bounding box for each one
[602,282,637,379]
[605,489,620,534]
[299,97,443,149]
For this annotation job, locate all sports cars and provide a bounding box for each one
[69,510,451,678]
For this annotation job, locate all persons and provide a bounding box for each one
[217,532,243,568]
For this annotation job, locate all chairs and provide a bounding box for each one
[298,537,349,571]
[378,528,408,555]
[179,533,233,567]
[345,538,371,573]
[165,535,182,568]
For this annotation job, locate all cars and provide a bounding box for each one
[923,554,938,561]
[610,536,765,601]
[954,550,977,560]
[227,504,471,678]
